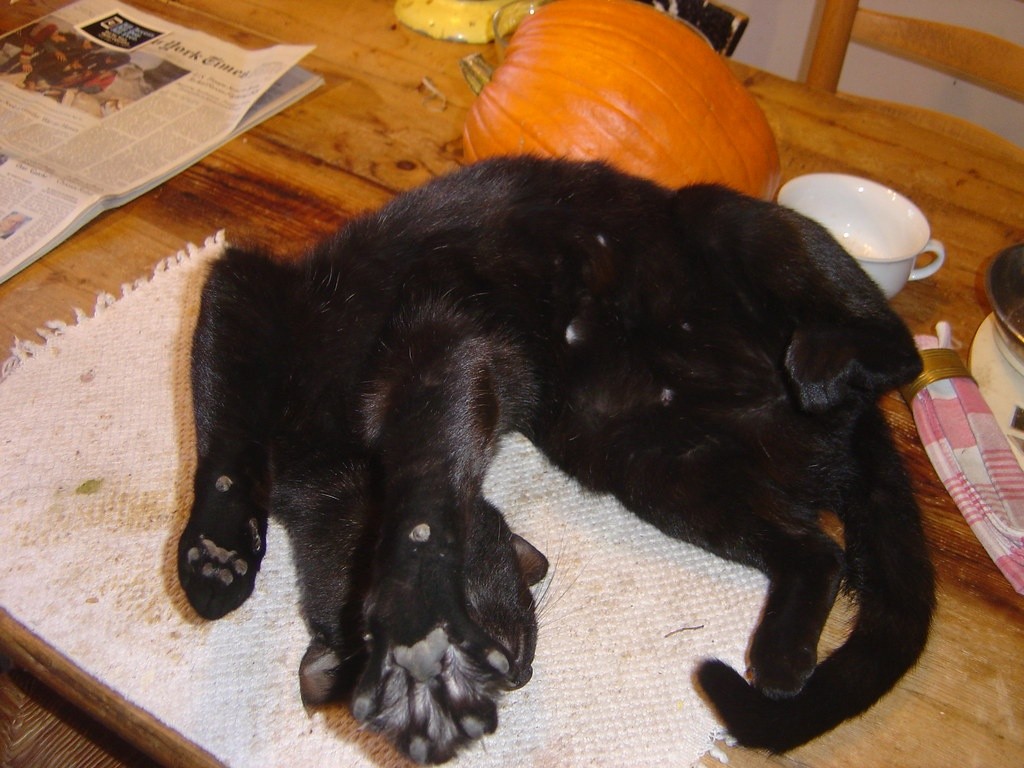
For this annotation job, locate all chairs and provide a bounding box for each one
[801,0,1024,104]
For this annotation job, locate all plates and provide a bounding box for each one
[983,242,1024,367]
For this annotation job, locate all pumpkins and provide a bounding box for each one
[461,0,782,203]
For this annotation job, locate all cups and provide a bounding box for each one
[775,170,945,310]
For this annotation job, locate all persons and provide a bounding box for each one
[0,23,131,97]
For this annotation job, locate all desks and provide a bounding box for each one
[0,0,1024,768]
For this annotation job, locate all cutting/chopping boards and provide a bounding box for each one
[966,314,1023,478]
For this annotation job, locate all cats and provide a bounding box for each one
[177,157,937,767]
